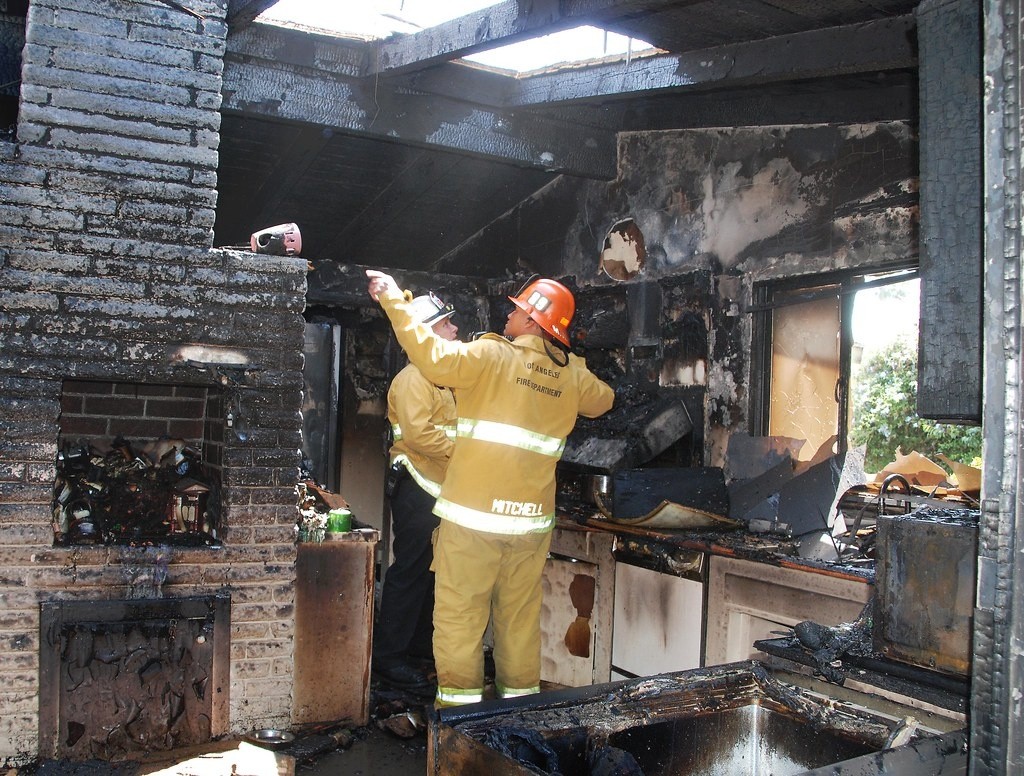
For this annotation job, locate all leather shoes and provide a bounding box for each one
[373,663,429,688]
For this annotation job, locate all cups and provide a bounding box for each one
[329,508,351,532]
[250,223,302,257]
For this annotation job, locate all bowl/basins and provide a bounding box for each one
[244,730,295,750]
[593,474,668,524]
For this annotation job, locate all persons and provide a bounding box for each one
[372,290,458,688]
[365,269,614,712]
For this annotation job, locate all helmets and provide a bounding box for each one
[507,279,575,350]
[401,290,456,354]
